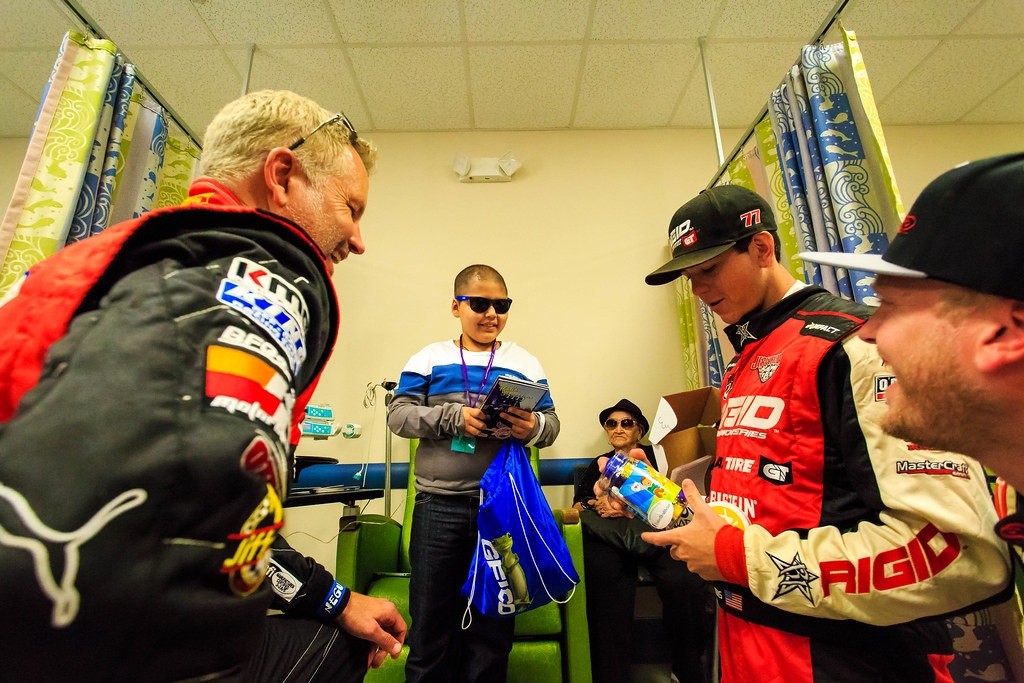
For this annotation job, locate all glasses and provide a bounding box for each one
[604,418,640,430]
[456,295,512,315]
[288,112,357,150]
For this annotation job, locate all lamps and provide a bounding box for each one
[451,151,522,183]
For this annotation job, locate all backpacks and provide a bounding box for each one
[459,438,581,630]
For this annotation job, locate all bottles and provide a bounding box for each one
[598,450,694,533]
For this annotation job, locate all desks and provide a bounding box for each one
[284,488,385,507]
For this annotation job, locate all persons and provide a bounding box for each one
[792,148,1024,683]
[0,88,407,682]
[573,397,659,683]
[388,264,561,682]
[597,184,1015,683]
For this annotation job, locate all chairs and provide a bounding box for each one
[335,437,593,683]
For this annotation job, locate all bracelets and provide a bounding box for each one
[580,498,593,510]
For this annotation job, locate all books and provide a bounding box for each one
[474,375,549,440]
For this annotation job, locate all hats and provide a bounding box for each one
[598,399,649,443]
[645,185,777,285]
[799,152,1024,302]
